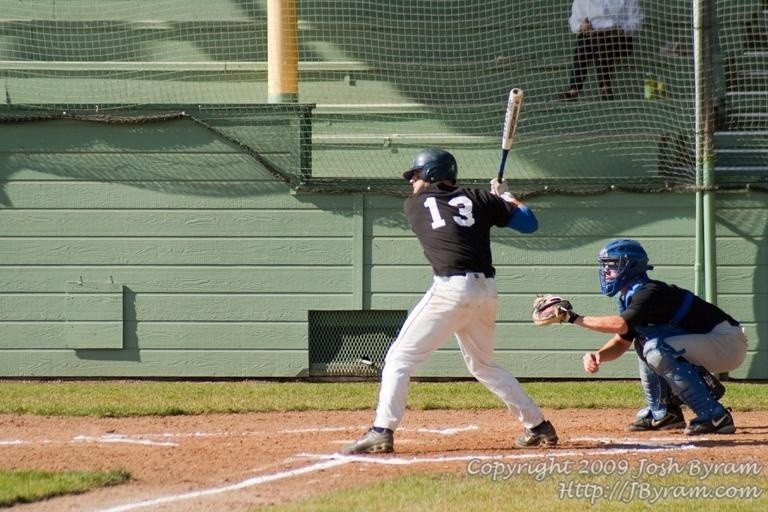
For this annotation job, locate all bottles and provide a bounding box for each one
[644,74,664,100]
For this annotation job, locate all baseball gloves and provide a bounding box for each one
[531,294,578,325]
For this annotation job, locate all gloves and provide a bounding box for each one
[489,177,517,204]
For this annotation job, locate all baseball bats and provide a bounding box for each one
[496,88,524,183]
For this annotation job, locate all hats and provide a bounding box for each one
[402,146,459,180]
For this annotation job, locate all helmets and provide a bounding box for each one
[596,239,649,297]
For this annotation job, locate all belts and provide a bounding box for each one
[447,270,495,279]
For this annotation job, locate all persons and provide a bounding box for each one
[339,149,559,456]
[554,0,643,101]
[531,238,749,436]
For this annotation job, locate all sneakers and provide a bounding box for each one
[340,425,395,455]
[513,419,559,448]
[556,91,579,103]
[683,406,738,435]
[600,91,615,102]
[623,407,687,433]
